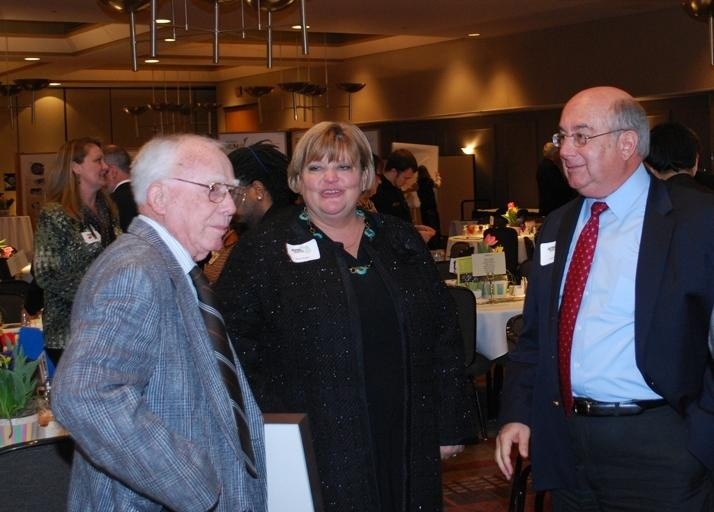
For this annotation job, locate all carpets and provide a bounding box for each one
[189,265,259,480]
[557,201,609,416]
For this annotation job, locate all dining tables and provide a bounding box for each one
[0,336,43,447]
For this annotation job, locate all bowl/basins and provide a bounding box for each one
[299,204,376,277]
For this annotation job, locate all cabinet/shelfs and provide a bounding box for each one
[463,224,535,240]
[21,313,30,325]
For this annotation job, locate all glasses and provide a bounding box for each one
[243,31,366,126]
[0,26,50,130]
[122,66,221,139]
[126,1,312,70]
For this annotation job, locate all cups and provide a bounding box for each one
[169,177,248,209]
[551,129,628,149]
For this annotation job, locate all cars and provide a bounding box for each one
[431,249,443,262]
[0,323,21,355]
[477,242,488,253]
[444,276,527,300]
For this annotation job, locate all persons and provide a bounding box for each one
[49,134,267,512]
[369,148,437,244]
[643,120,714,195]
[32,135,121,365]
[417,165,442,247]
[214,139,300,229]
[212,119,473,510]
[536,142,565,216]
[100,143,136,235]
[355,150,382,212]
[492,86,714,511]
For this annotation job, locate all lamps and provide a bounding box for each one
[501,312,555,512]
[484,226,518,273]
[0,279,33,324]
[445,285,492,442]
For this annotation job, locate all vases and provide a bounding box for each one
[502,200,519,224]
